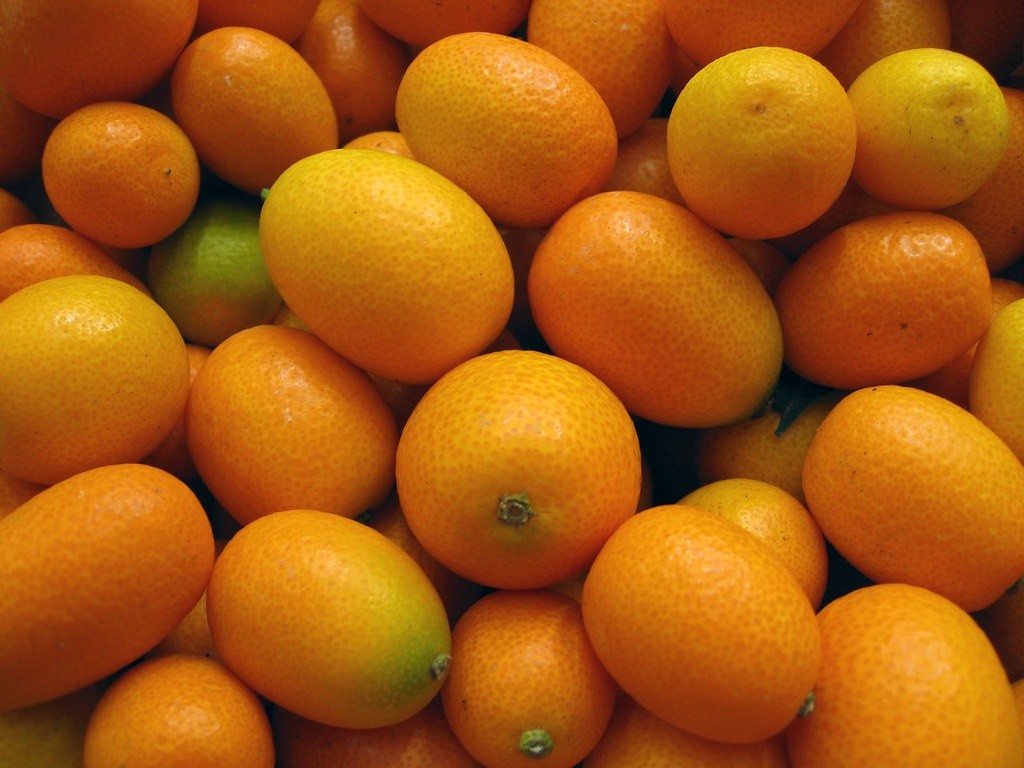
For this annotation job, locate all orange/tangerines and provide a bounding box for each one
[0,0,1024,768]
